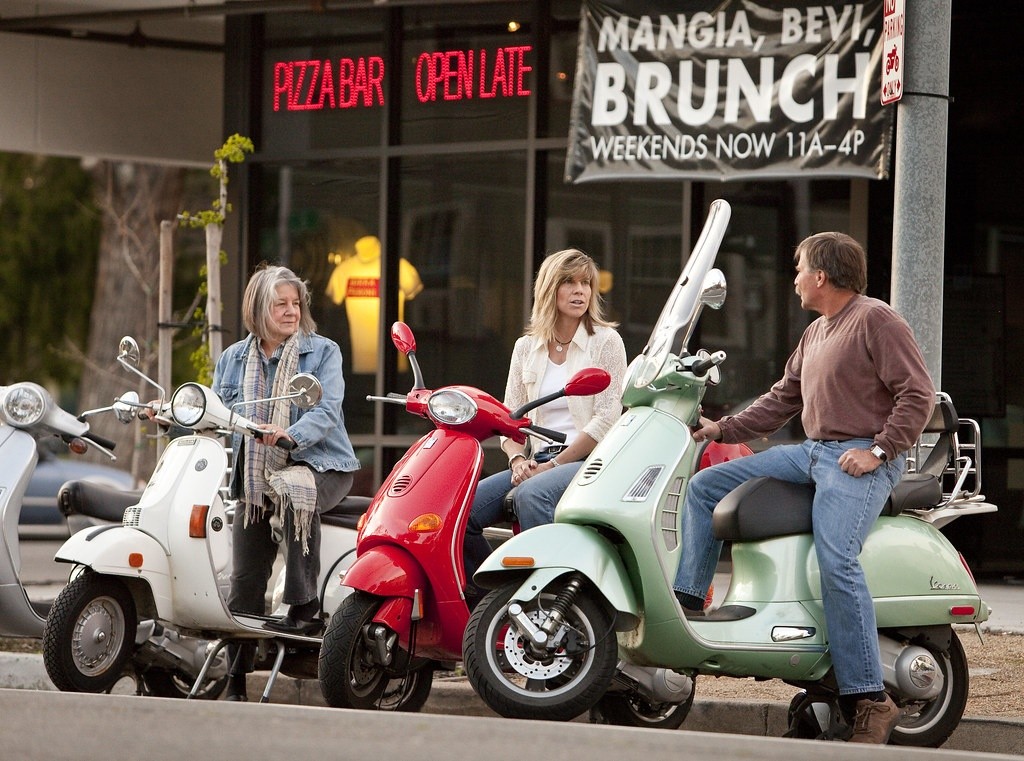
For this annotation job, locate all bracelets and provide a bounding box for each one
[550,459,560,467]
[508,454,527,469]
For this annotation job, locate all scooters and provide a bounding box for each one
[457,196,998,751]
[316,322,755,710]
[0,379,143,642]
[37,333,377,704]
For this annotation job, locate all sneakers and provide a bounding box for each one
[681,605,705,616]
[848,692,900,745]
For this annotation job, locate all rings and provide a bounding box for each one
[514,474,518,478]
[272,429,277,434]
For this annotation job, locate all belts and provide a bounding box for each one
[541,446,567,453]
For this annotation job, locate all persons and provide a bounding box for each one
[668,232,937,747]
[463,248,627,603]
[145,264,361,701]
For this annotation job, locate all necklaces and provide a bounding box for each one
[555,337,572,352]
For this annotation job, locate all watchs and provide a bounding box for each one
[870,445,887,462]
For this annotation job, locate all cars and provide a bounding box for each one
[16,435,147,541]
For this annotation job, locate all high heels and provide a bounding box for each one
[261,612,322,636]
[226,686,249,701]
